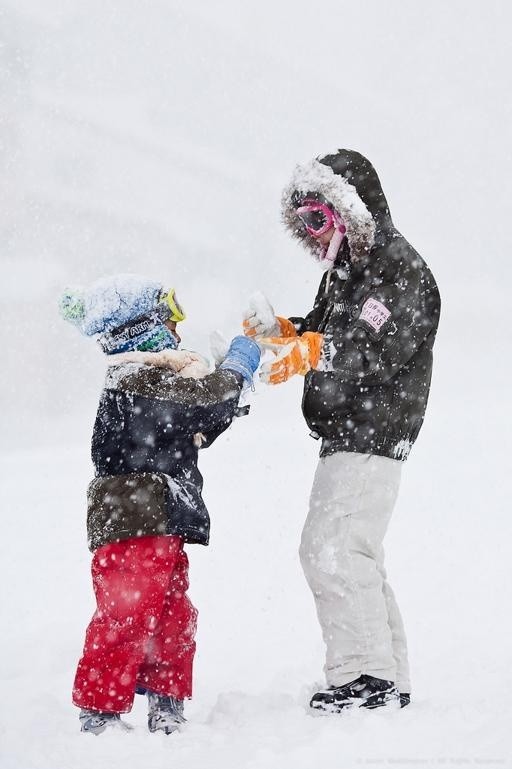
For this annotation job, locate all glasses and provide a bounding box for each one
[110,286,186,341]
[294,202,334,236]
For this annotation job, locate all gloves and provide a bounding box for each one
[257,330,324,386]
[242,309,297,344]
[218,335,262,392]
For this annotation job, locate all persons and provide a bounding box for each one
[240,148,443,711]
[58,278,263,731]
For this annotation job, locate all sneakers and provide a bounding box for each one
[308,674,400,715]
[78,693,188,737]
